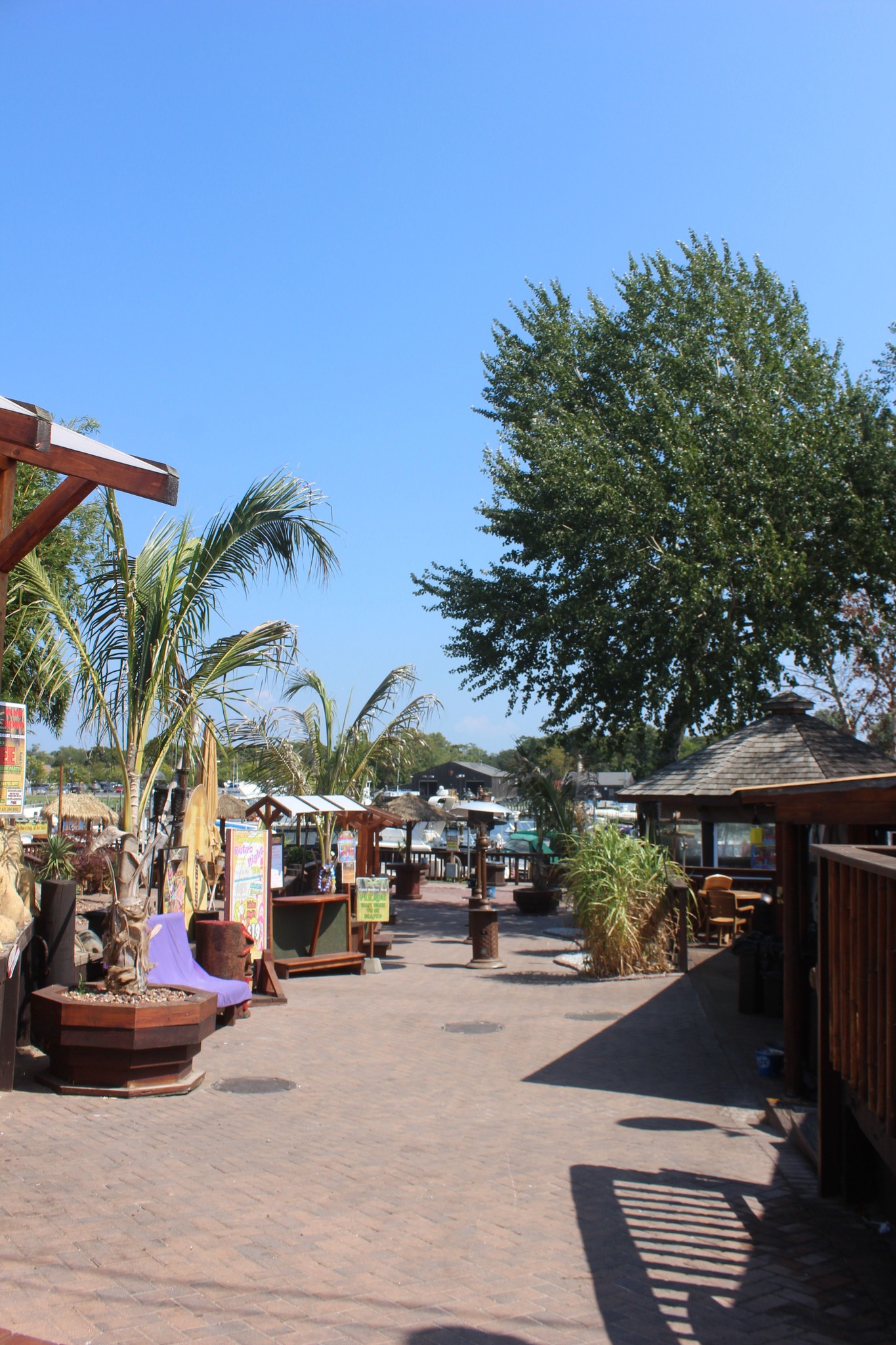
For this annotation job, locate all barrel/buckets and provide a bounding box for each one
[487,886,495,898]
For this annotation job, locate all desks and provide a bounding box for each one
[697,889,762,945]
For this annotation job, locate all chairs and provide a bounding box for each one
[697,874,764,950]
